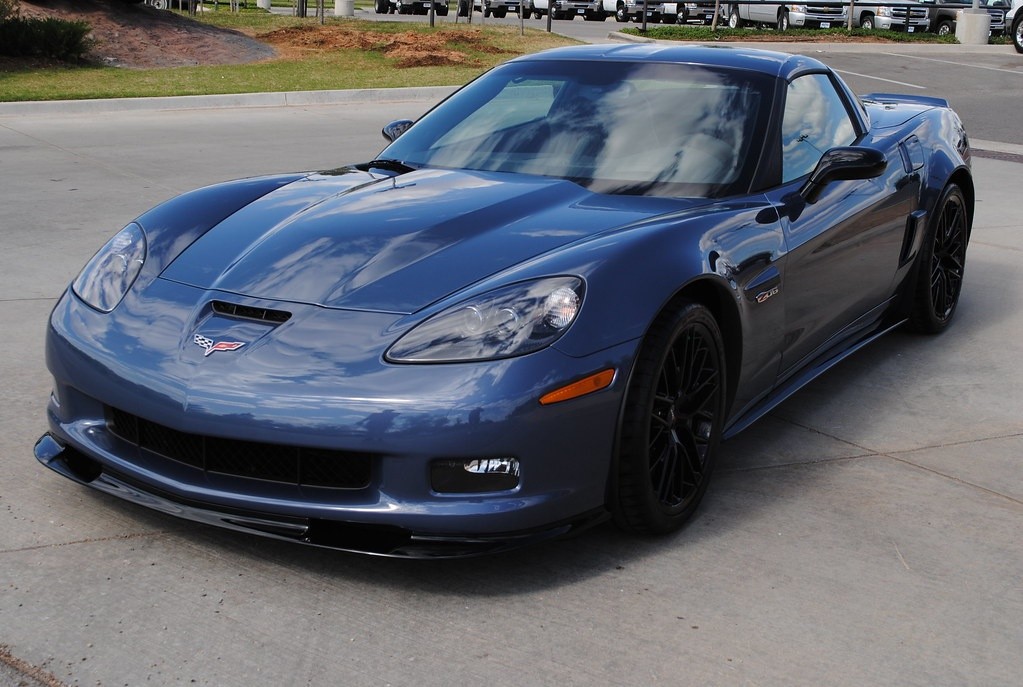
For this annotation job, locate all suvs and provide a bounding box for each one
[373,0,1012,36]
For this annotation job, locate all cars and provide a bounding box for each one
[1004,0,1023,54]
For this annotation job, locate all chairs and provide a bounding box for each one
[533,81,663,170]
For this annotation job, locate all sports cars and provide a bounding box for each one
[33,39,976,564]
